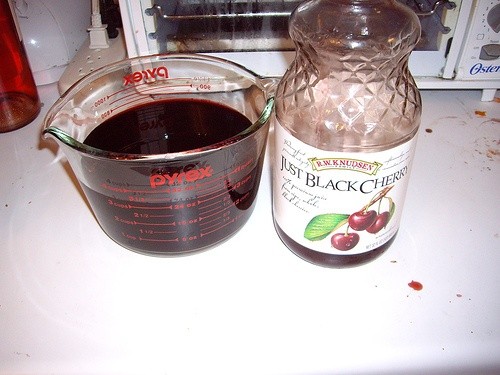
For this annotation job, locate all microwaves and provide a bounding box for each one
[119,0,500,101]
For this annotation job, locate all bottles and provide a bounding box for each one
[0,0,41,132]
[272,0,422,269]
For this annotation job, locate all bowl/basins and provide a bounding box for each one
[41,54,282,254]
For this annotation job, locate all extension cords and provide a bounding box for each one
[59,25,127,96]
